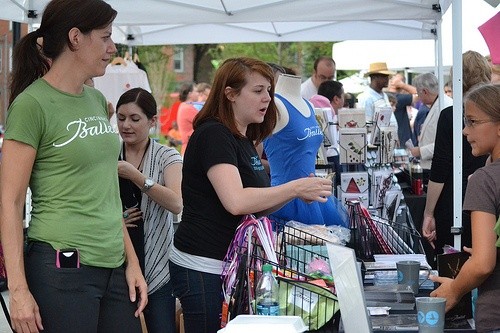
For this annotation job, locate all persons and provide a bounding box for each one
[430,84,500,333]
[116,87,183,333]
[254,62,452,195]
[254,74,325,232]
[0,0,148,333]
[167,82,211,155]
[168,58,334,332]
[421,50,492,333]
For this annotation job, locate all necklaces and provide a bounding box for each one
[123,141,150,169]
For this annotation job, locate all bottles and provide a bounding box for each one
[256,264,280,316]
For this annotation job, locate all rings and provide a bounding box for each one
[123,212,129,218]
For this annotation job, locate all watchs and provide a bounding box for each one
[141,177,155,192]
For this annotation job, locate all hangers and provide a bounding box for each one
[109,45,145,72]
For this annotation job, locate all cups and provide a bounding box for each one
[396,260,430,288]
[415,297,447,333]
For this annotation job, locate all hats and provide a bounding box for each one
[363,62,396,77]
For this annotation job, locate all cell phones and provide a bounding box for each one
[56,248,80,269]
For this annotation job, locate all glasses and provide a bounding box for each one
[464,116,495,126]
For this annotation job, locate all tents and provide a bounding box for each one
[0,0,461,252]
[333,0,492,85]
